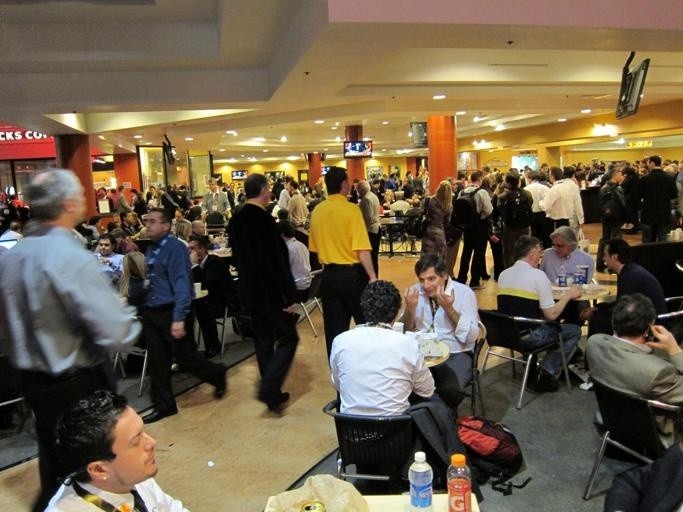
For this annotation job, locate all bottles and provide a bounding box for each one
[407,450,434,512]
[557,265,567,287]
[573,264,584,285]
[444,453,472,512]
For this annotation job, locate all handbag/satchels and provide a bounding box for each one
[595,184,628,221]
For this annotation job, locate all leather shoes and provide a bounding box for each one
[211,361,227,398]
[141,404,178,424]
[204,341,221,359]
[525,368,560,392]
[257,390,289,410]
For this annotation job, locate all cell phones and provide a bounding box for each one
[645,328,659,343]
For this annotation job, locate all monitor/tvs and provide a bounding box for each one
[162,133,176,165]
[412,122,429,148]
[343,140,372,158]
[98,199,111,214]
[232,170,248,180]
[320,166,336,176]
[614,58,650,121]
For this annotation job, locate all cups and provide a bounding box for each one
[193,282,201,297]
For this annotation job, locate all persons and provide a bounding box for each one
[0,168,142,502]
[585,293,682,451]
[38,390,192,512]
[1,154,682,426]
[351,142,364,152]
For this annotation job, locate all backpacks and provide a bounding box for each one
[454,413,532,497]
[452,185,483,230]
[402,199,431,239]
[500,187,531,227]
